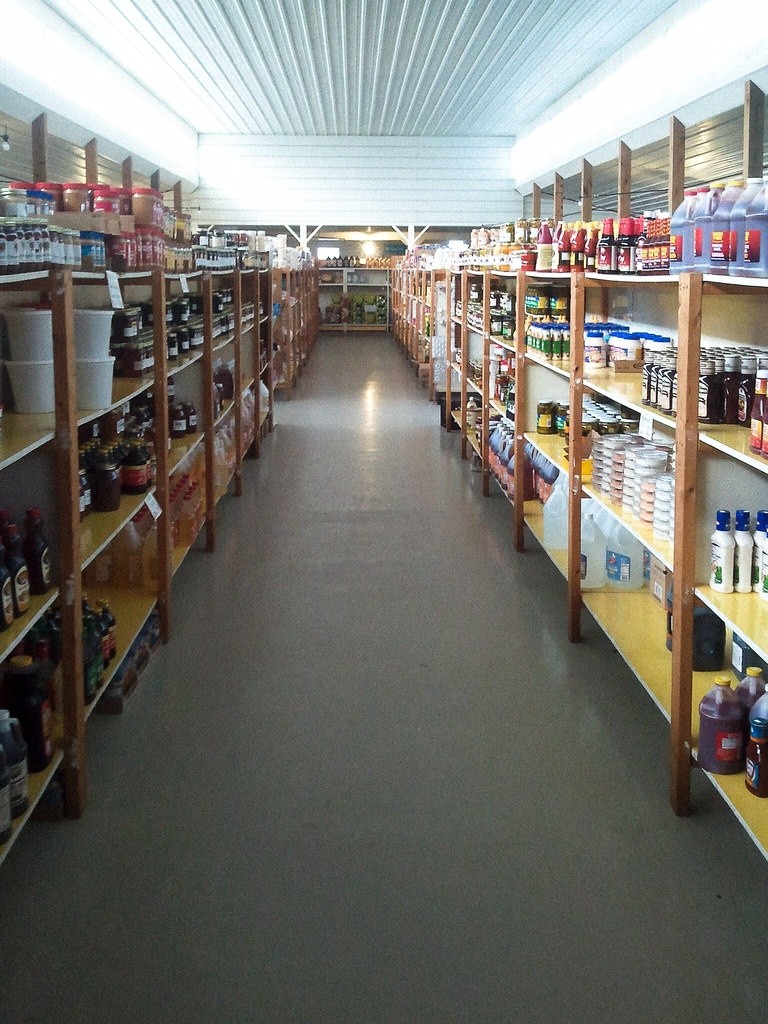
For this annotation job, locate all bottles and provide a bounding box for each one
[0,182,392,847]
[444,178,768,791]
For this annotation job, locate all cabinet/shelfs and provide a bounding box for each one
[0,79,768,875]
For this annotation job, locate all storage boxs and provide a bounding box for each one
[648,553,703,611]
